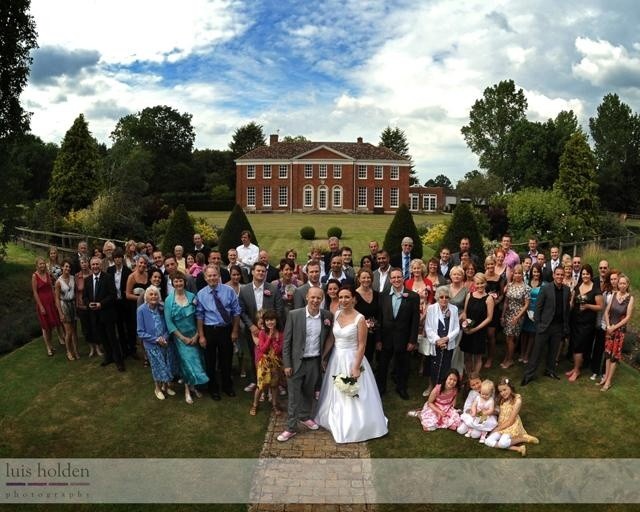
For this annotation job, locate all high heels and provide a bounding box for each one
[518,354,523,362]
[523,354,528,363]
[500,360,513,368]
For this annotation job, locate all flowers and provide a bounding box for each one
[365,317,378,335]
[488,291,499,300]
[332,365,364,400]
[460,318,475,333]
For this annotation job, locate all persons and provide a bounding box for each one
[354,269,379,364]
[320,256,354,285]
[248,309,288,417]
[360,254,373,272]
[408,368,462,433]
[323,236,340,276]
[125,256,152,368]
[499,264,531,369]
[456,373,540,456]
[313,286,389,445]
[521,256,532,286]
[106,248,141,359]
[224,265,244,294]
[463,259,477,282]
[30,257,61,357]
[45,237,115,270]
[536,252,554,283]
[422,287,461,396]
[195,263,240,401]
[483,256,504,370]
[54,260,81,362]
[246,248,279,286]
[373,268,421,401]
[389,236,413,281]
[125,232,210,273]
[565,264,605,382]
[500,235,518,270]
[438,247,454,281]
[224,248,249,274]
[561,253,578,292]
[135,285,180,401]
[451,237,479,268]
[526,237,540,266]
[237,261,286,393]
[595,272,635,393]
[339,247,356,284]
[589,268,623,381]
[594,259,611,295]
[269,258,304,310]
[82,256,128,374]
[235,232,260,275]
[494,248,511,286]
[462,273,494,372]
[520,266,572,386]
[444,266,468,382]
[404,259,434,377]
[277,287,334,443]
[571,255,582,282]
[73,256,105,358]
[425,257,447,286]
[372,249,395,295]
[368,240,380,273]
[275,248,304,283]
[164,270,210,404]
[542,245,562,272]
[301,247,326,283]
[324,279,342,314]
[292,260,331,313]
[517,263,543,364]
[162,255,196,301]
[459,249,472,260]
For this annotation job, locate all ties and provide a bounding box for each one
[94,277,98,297]
[211,290,228,323]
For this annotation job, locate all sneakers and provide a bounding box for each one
[479,432,487,444]
[259,393,265,402]
[464,428,471,438]
[523,434,539,444]
[243,382,256,392]
[594,378,605,386]
[278,386,287,396]
[568,372,580,382]
[300,419,319,430]
[408,411,418,418]
[589,372,597,381]
[520,444,526,457]
[600,384,612,392]
[276,431,296,442]
[314,389,320,400]
[565,368,574,375]
[422,386,433,397]
[95,345,104,357]
[88,347,94,358]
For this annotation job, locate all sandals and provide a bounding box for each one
[271,404,282,416]
[161,386,176,396]
[155,389,165,400]
[143,359,148,368]
[249,404,256,416]
[51,346,57,353]
[184,392,193,405]
[74,350,80,360]
[66,351,75,361]
[191,387,202,398]
[47,348,53,356]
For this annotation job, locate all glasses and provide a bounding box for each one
[439,296,449,299]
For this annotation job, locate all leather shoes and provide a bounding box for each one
[209,389,221,401]
[100,358,112,367]
[396,387,409,400]
[222,387,236,397]
[543,371,561,380]
[520,376,532,386]
[117,361,125,372]
